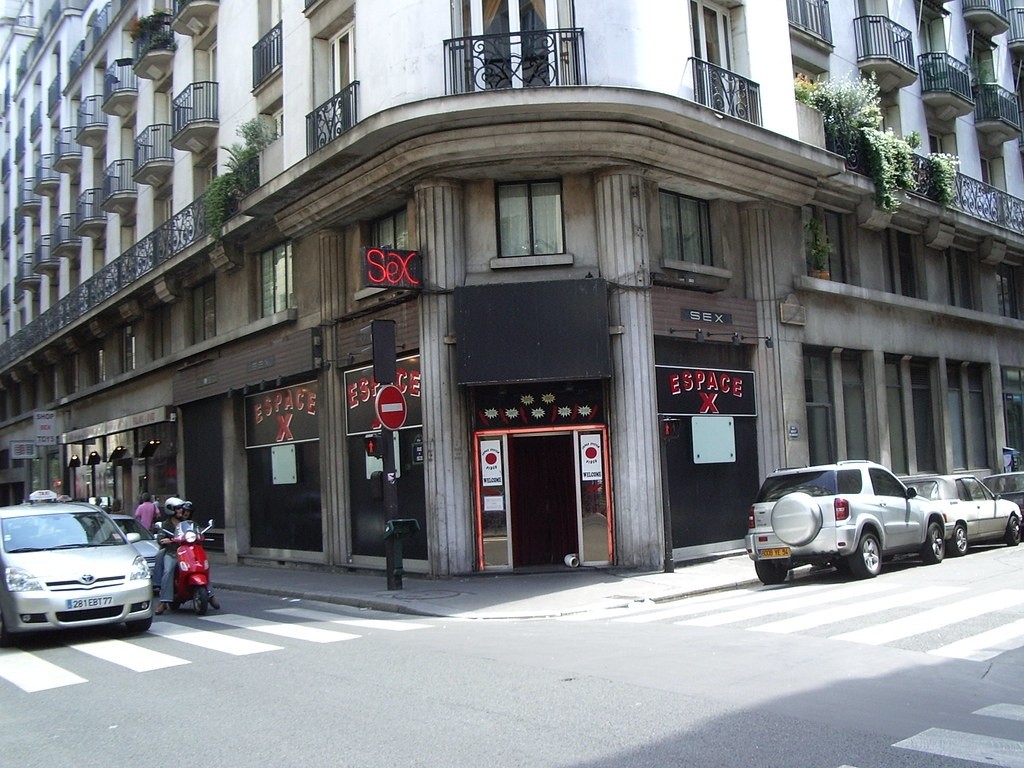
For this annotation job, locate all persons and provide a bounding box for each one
[95,496,102,506]
[109,499,127,514]
[152,497,220,615]
[135,492,160,535]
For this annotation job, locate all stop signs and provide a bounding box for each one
[377,385,407,431]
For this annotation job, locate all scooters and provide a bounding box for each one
[154,519,217,615]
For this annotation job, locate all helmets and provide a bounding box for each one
[183,501,192,511]
[164,497,187,516]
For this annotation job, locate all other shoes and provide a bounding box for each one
[155,603,167,614]
[209,596,220,609]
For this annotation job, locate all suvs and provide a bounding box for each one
[744,458,947,585]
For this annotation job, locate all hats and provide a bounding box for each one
[142,493,150,499]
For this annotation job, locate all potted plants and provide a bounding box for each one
[805,216,836,279]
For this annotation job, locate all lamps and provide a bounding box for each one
[141,440,161,459]
[108,446,127,462]
[87,451,102,465]
[69,455,81,469]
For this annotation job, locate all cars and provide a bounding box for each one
[983,472,1024,539]
[0,490,154,645]
[109,513,162,597]
[896,473,1022,558]
